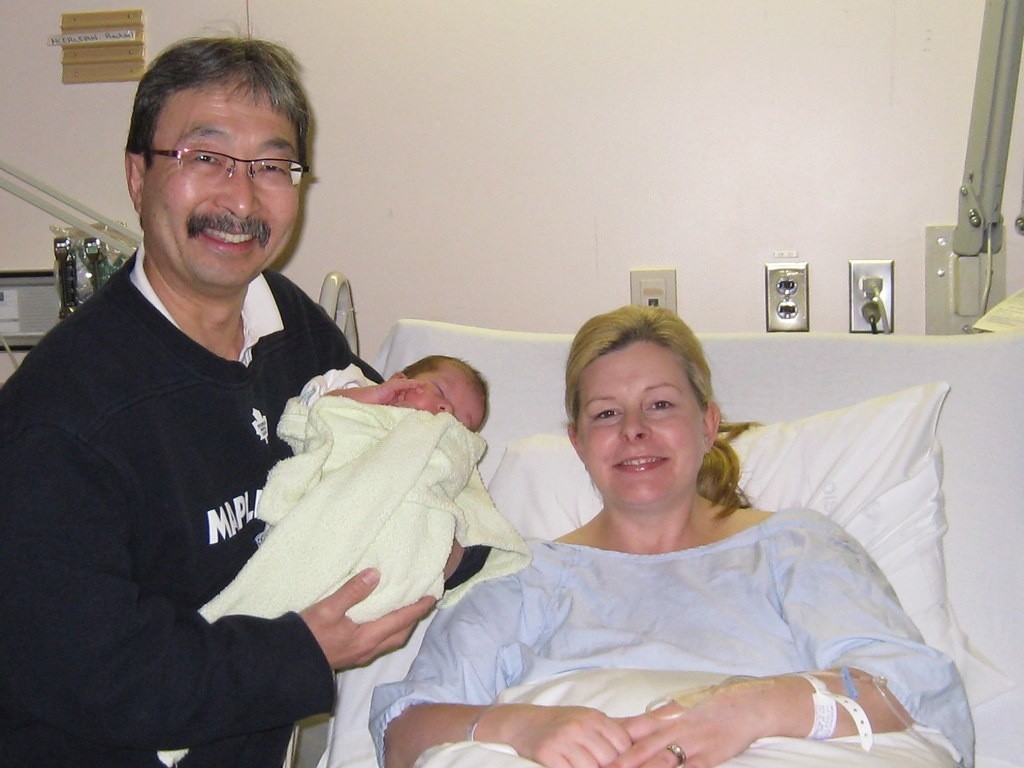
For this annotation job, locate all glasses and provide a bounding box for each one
[147,149,309,191]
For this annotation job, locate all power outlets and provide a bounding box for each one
[630,268,678,316]
[763,261,810,333]
[850,259,894,333]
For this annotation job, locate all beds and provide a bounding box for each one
[293,320,1024,768]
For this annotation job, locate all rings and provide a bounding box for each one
[665,742,688,763]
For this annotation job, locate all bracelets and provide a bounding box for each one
[792,672,874,751]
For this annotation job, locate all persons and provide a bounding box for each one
[1,37,975,768]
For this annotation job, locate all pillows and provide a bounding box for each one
[491,379,966,677]
